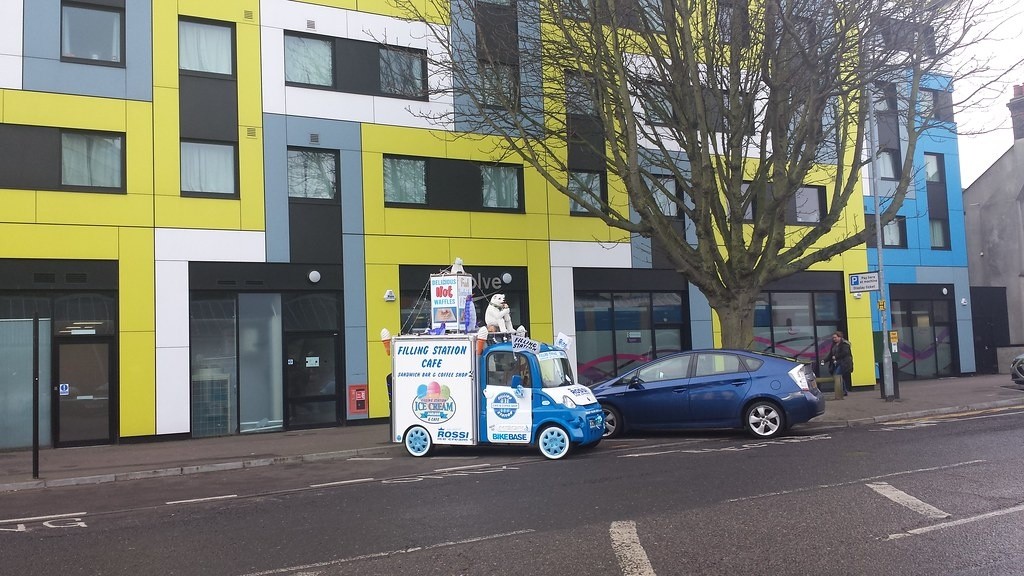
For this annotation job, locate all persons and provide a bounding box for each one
[821,330,853,396]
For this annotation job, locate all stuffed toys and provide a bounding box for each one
[485,293,516,333]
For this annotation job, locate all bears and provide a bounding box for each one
[485,293,517,334]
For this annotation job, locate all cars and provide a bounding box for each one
[593,347,826,439]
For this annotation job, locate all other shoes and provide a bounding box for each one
[843,392,847,396]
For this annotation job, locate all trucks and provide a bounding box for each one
[390,331,606,460]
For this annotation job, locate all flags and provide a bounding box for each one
[555,333,572,350]
[511,334,540,354]
